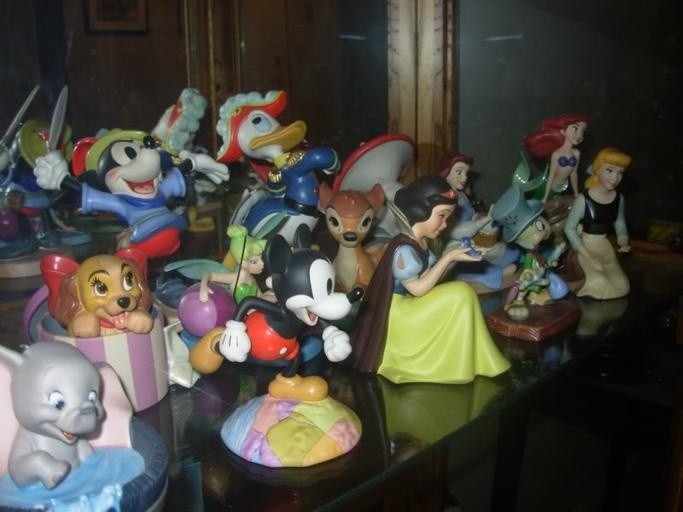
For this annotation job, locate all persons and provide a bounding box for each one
[529,115,593,269]
[564,146,629,299]
[199,225,278,319]
[0,81,633,511]
[437,151,522,287]
[346,177,511,384]
